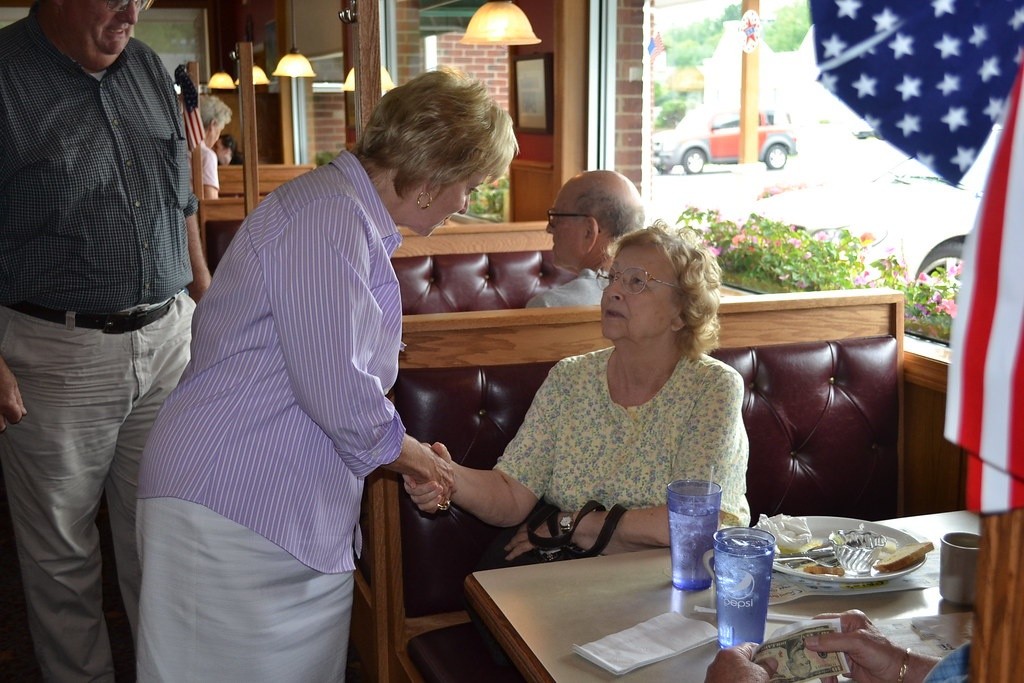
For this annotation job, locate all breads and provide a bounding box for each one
[873,542,934,572]
[803,566,844,576]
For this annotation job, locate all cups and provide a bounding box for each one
[666,479,722,592]
[713,527,777,650]
[827,529,886,577]
[939,532,981,605]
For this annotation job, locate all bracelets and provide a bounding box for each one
[898,648,911,683]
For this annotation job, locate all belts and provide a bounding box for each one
[0,296,174,335]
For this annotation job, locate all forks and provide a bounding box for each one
[773,557,841,568]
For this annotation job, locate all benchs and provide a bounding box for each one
[172,162,902,683]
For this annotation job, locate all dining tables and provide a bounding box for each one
[462,512,1024,683]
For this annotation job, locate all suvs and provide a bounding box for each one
[652,103,797,174]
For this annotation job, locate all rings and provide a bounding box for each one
[436,500,451,511]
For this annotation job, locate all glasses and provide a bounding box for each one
[105,0,156,13]
[546,208,602,233]
[596,266,681,296]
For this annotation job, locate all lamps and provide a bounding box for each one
[207,0,546,93]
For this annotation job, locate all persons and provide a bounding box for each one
[186,94,232,199]
[0,0,212,683]
[134,69,520,683]
[526,170,643,309]
[217,134,244,165]
[401,219,749,566]
[704,608,969,682]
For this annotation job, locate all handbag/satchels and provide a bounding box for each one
[459,499,627,668]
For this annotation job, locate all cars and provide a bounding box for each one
[753,127,1003,286]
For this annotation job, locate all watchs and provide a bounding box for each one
[559,510,574,546]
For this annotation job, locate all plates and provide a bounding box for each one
[752,516,927,583]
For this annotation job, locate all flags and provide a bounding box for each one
[174,63,204,149]
[808,0,1024,516]
